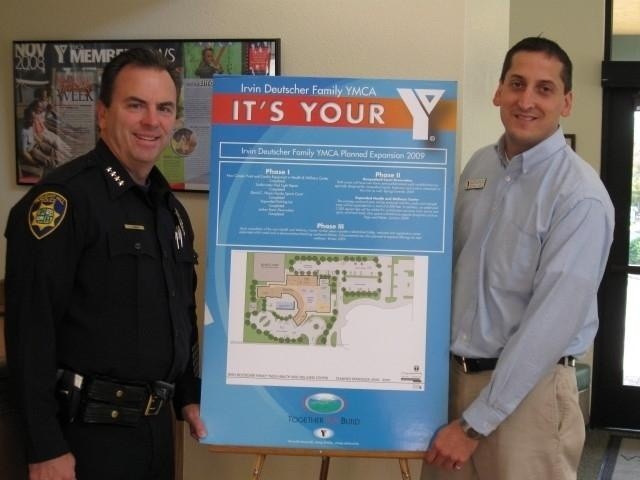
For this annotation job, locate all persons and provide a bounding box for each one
[247,39,272,77]
[4,47,207,480]
[194,48,224,78]
[18,97,88,170]
[424,37,616,480]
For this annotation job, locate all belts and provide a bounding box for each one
[450,351,576,374]
[58,369,169,416]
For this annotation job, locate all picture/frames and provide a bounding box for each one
[12,39,282,193]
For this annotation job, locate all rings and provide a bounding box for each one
[455,464,461,470]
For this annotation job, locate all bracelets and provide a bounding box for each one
[460,415,484,440]
